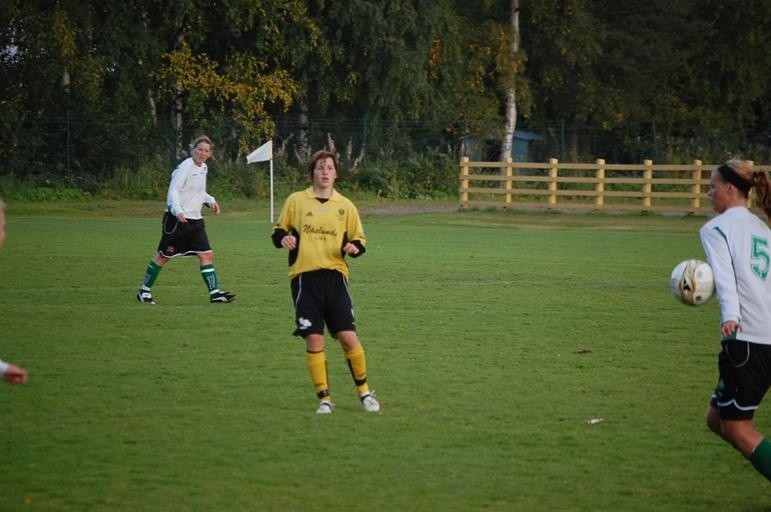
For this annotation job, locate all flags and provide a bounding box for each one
[245,139,273,164]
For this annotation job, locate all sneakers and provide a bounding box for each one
[316,400,335,414]
[359,390,380,412]
[138,289,156,305]
[210,291,235,302]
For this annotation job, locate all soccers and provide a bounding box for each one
[671,260,715,306]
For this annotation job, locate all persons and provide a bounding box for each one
[697,158,769,484]
[0,200,28,386]
[269,149,380,413]
[136,135,237,304]
